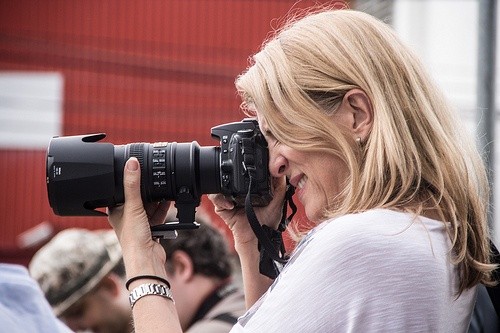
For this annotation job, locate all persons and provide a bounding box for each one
[0,210,284,333]
[105,11,496,333]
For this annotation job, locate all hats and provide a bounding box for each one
[29,227,125,317]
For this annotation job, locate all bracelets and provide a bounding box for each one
[125,274,170,290]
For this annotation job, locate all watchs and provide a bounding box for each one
[129,283,175,310]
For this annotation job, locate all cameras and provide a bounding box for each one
[44,117,273,241]
